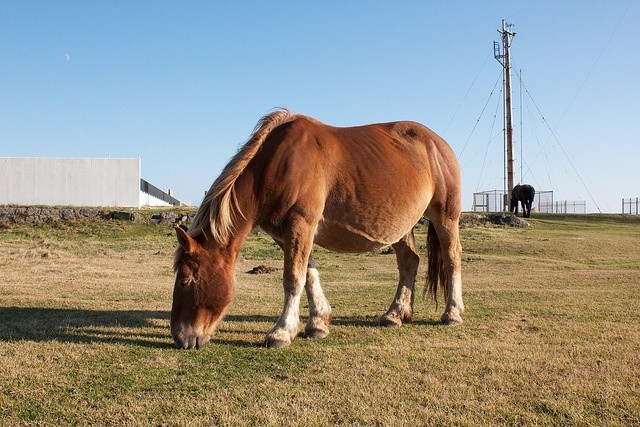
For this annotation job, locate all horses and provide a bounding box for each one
[171,107,465,350]
[510,184,535,218]
